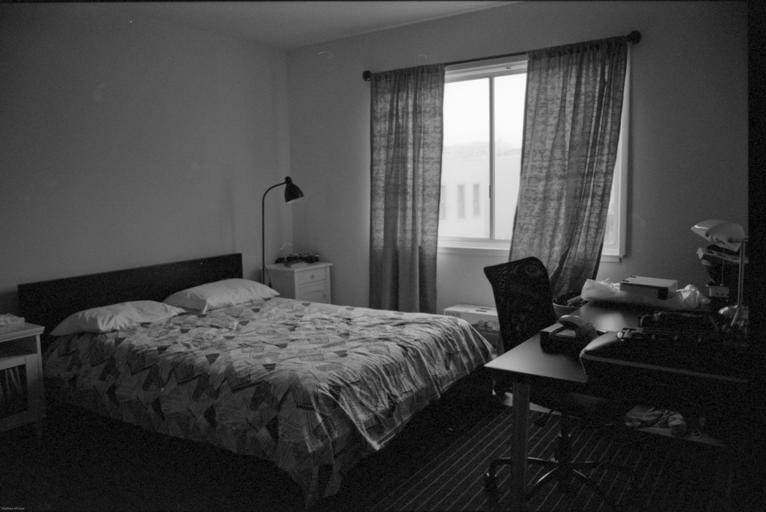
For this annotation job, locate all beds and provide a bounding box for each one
[17,251,494,509]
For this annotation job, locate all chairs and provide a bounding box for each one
[483,256,645,511]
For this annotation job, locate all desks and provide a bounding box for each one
[482,295,766,512]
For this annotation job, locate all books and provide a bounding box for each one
[697,246,750,266]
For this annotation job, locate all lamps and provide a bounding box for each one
[262,176,305,284]
[691,220,746,328]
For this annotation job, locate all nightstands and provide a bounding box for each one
[263,262,334,304]
[0,322,47,435]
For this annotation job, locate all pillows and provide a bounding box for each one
[164,278,281,315]
[50,300,187,337]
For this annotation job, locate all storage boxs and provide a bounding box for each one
[443,303,500,353]
